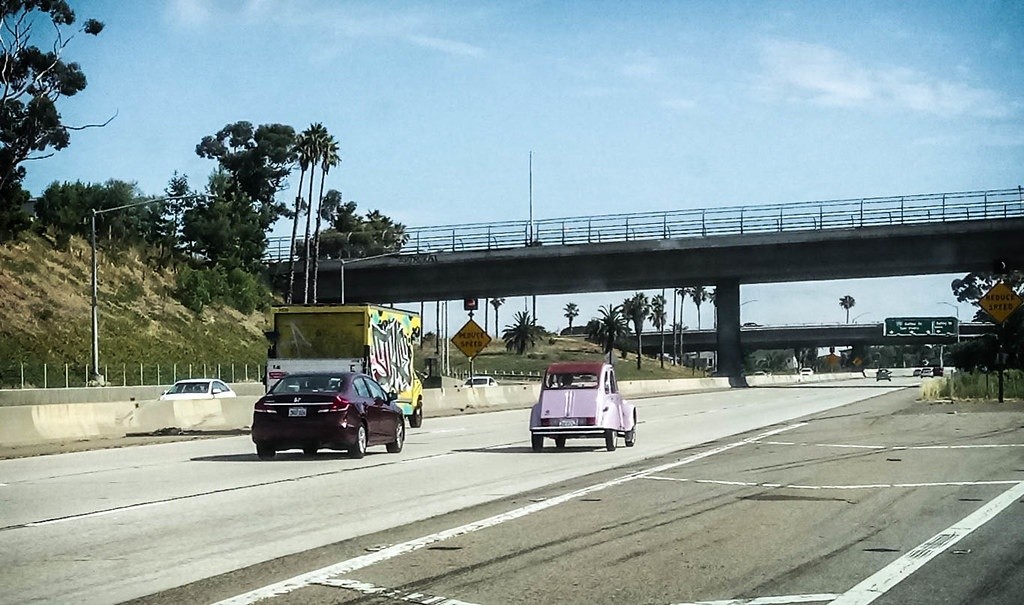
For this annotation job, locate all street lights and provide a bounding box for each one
[937,301,959,319]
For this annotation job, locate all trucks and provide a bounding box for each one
[260,302,424,428]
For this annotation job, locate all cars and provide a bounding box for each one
[156,377,238,401]
[753,370,766,376]
[461,375,498,389]
[875,369,892,381]
[799,367,814,377]
[932,367,943,377]
[251,370,406,462]
[913,369,921,377]
[920,368,933,378]
[528,361,638,452]
